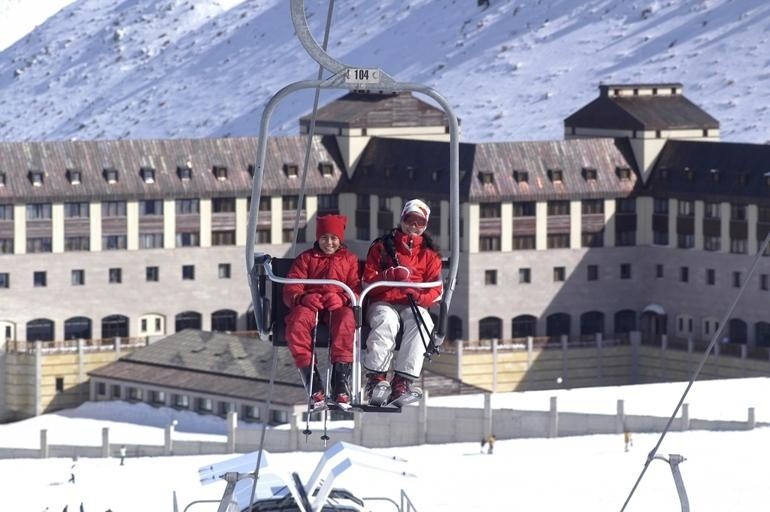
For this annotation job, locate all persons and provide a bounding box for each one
[66,464,78,485]
[480,437,487,454]
[355,196,443,406]
[487,435,496,454]
[119,445,127,466]
[285,212,353,407]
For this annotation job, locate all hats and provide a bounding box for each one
[401,199,432,221]
[315,215,346,242]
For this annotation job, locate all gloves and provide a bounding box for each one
[301,292,344,312]
[388,265,421,299]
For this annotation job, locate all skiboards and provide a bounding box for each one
[310,401,361,417]
[368,380,424,410]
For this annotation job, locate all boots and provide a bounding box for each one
[297,361,324,403]
[330,360,353,404]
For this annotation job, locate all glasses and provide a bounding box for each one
[403,212,427,229]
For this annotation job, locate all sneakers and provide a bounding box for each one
[365,370,388,402]
[389,373,414,405]
